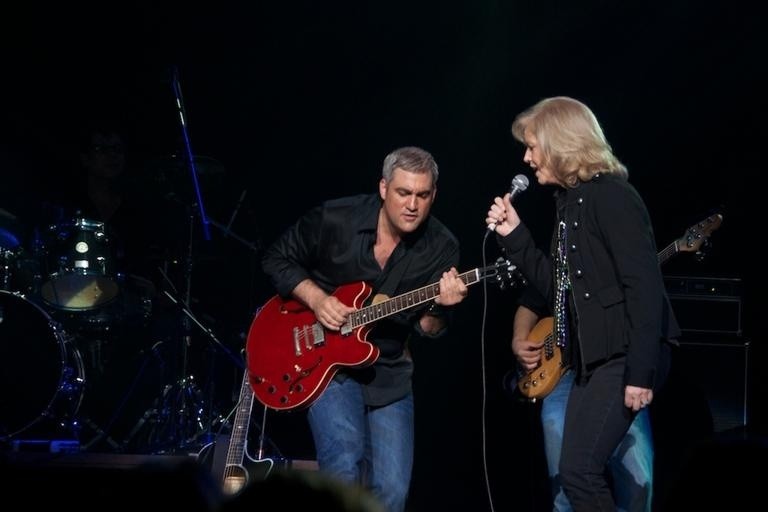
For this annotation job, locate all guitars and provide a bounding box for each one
[516,211,723,403]
[245,257,528,413]
[197,368,274,497]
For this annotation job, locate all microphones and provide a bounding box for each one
[487,174,529,232]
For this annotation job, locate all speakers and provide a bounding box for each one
[672,339,750,441]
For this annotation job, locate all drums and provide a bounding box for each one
[0,243,39,305]
[0,290,87,441]
[31,216,124,311]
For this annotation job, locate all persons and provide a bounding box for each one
[43,124,175,330]
[508,199,658,510]
[484,95,684,510]
[257,146,471,511]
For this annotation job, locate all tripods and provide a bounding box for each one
[114,218,230,457]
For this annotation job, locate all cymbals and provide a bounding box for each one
[116,272,155,291]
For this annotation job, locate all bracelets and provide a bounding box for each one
[427,301,447,317]
